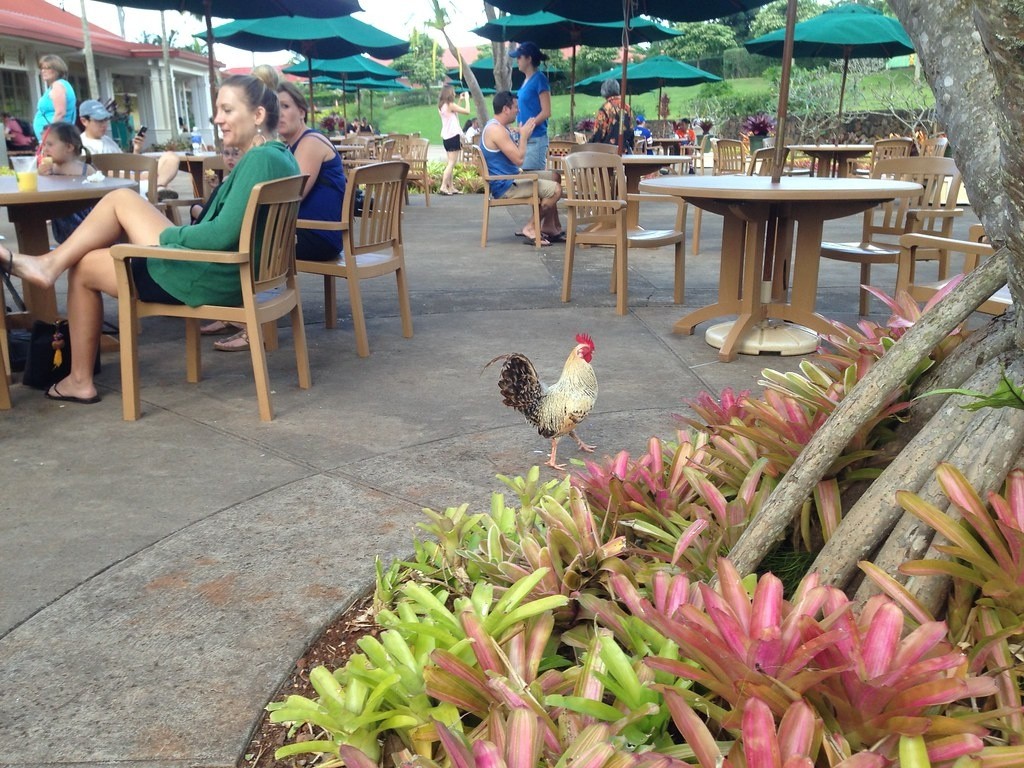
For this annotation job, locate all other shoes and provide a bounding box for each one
[689,168,695,174]
[145,184,178,201]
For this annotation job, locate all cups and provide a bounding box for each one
[9,156,38,193]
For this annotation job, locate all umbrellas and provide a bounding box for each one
[744,2,918,177]
[90,0,412,184]
[445,0,796,329]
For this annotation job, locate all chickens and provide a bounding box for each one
[480,332,599,471]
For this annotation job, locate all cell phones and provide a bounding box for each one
[138,125,147,137]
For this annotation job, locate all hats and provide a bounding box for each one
[636,114,647,124]
[79,99,113,120]
[508,41,542,60]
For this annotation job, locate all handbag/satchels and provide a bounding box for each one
[22,320,102,389]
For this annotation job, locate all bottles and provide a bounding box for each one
[191,127,203,156]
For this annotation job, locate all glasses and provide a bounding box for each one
[40,64,52,71]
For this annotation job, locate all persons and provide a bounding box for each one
[437,84,480,196]
[632,115,654,156]
[480,90,566,246]
[585,79,633,214]
[201,82,346,352]
[0,55,245,246]
[0,65,300,404]
[508,42,562,236]
[346,118,360,135]
[359,117,373,134]
[674,118,695,175]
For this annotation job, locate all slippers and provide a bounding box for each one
[44,382,101,404]
[450,189,462,194]
[201,321,242,335]
[515,231,567,246]
[0,249,26,311]
[214,329,251,351]
[439,189,453,196]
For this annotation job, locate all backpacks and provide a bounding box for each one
[602,100,635,153]
[6,118,33,137]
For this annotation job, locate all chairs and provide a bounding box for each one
[0,128,431,423]
[469,124,1012,363]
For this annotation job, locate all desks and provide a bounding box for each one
[578,155,692,248]
[0,174,139,351]
[788,145,874,178]
[653,138,687,175]
[143,151,218,198]
[637,174,924,363]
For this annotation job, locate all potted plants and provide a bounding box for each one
[697,118,712,153]
[744,114,773,155]
